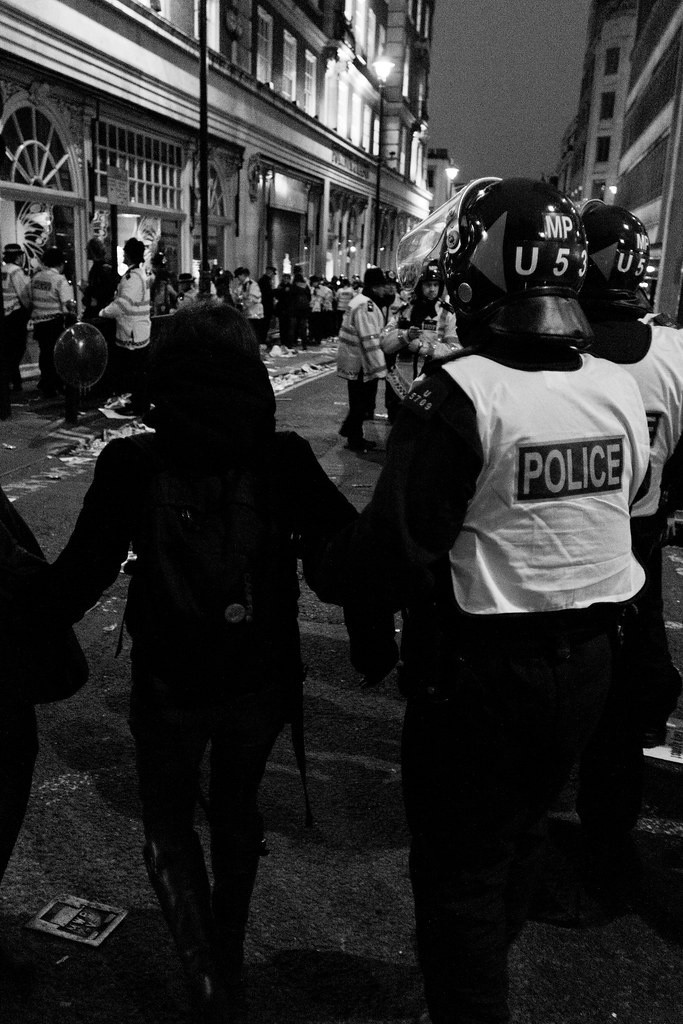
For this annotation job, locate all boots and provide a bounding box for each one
[142,828,261,991]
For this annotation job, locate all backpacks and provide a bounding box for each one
[128,427,293,634]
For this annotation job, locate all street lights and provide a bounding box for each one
[445,163,460,200]
[372,54,396,266]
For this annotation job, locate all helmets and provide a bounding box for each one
[443,179,587,319]
[583,204,650,301]
[415,270,445,300]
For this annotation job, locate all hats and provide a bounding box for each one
[385,270,401,292]
[362,268,387,287]
[2,244,25,257]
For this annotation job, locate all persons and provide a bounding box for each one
[353,173,648,1023]
[379,260,463,425]
[47,295,397,1024]
[335,268,387,449]
[576,198,683,763]
[0,236,363,419]
[364,264,427,422]
[0,489,88,882]
